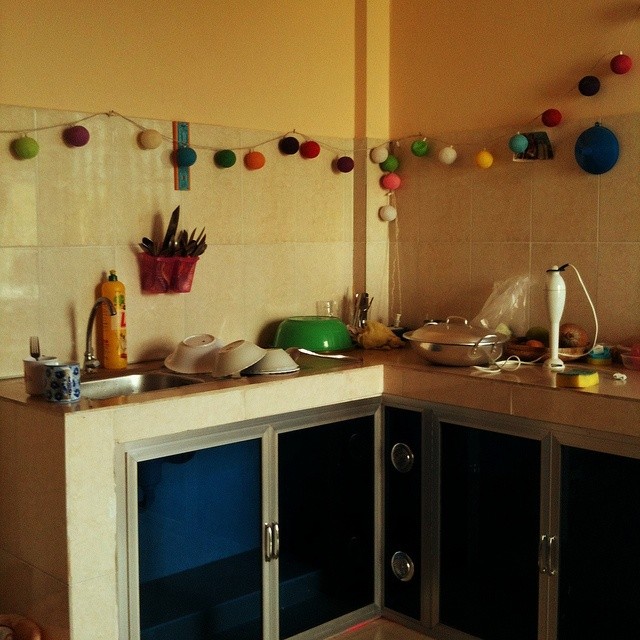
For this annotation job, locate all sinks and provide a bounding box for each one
[79,371,205,401]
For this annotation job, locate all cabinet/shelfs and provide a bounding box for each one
[424,399,639,639]
[121,391,380,640]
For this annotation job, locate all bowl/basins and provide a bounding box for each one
[164,334,223,374]
[240,347,300,375]
[212,339,267,378]
[620,352,640,370]
[270,315,360,351]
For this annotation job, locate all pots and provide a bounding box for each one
[402,316,509,366]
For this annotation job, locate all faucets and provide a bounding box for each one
[85,297,116,370]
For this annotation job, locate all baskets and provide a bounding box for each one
[143,253,199,294]
[502,337,593,362]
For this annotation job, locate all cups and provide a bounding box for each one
[23,355,58,395]
[43,360,80,404]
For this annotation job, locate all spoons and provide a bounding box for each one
[168,235,178,255]
[142,237,153,247]
[192,244,208,257]
[184,240,198,256]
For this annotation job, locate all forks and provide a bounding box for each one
[30,336,41,361]
[138,243,155,256]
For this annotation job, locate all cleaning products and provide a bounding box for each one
[101,268,127,369]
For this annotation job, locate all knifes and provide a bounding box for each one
[198,234,207,245]
[163,205,180,249]
[178,231,182,245]
[183,229,188,243]
[188,227,197,241]
